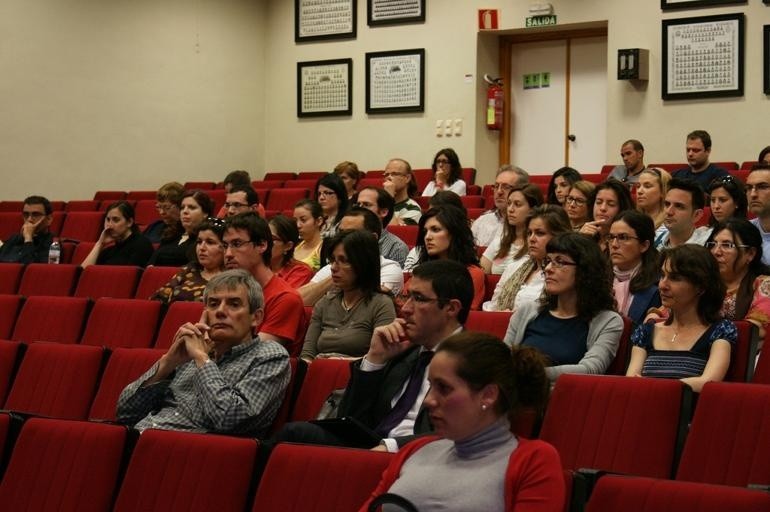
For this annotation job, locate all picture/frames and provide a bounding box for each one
[661,0,747,12]
[364,48,426,115]
[367,0,426,27]
[762,24,770,94]
[294,0,357,43]
[661,13,745,100]
[297,57,351,119]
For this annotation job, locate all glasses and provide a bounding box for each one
[24,213,48,219]
[156,160,754,302]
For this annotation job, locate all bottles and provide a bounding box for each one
[47,236,62,264]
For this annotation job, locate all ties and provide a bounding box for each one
[376,350,439,438]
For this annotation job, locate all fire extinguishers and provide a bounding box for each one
[484,73,505,130]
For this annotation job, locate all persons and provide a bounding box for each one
[114,269,291,443]
[359,330,566,511]
[274,258,476,455]
[0,195,64,265]
[84,127,770,391]
